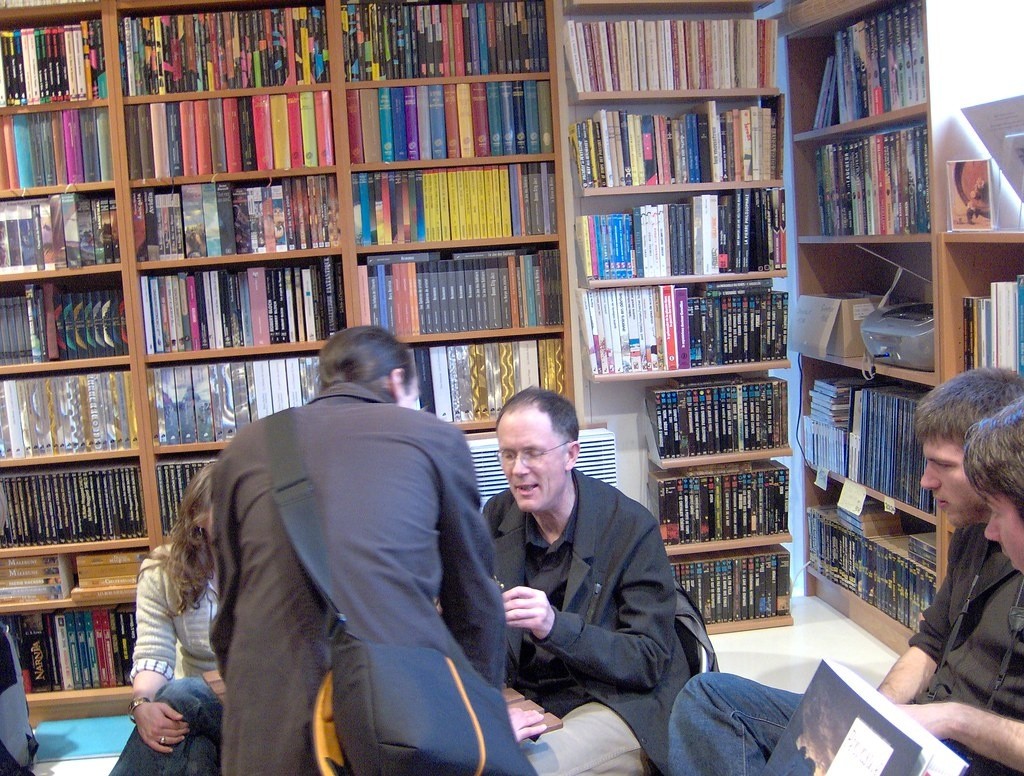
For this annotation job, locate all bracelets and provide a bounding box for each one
[128,697,150,724]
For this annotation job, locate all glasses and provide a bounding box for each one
[495,442,569,466]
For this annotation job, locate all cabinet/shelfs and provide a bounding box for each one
[0,0,1024,728]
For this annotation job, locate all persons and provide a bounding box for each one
[215,327,538,776]
[105,463,222,776]
[964,396,1024,572]
[482,386,692,776]
[668,367,1024,776]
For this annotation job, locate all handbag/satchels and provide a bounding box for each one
[310,637,538,775]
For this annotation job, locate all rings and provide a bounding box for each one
[160,736,165,744]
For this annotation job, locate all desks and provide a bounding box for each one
[196,661,562,744]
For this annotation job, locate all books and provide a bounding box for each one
[759,658,971,776]
[801,3,1024,634]
[0,0,789,693]
